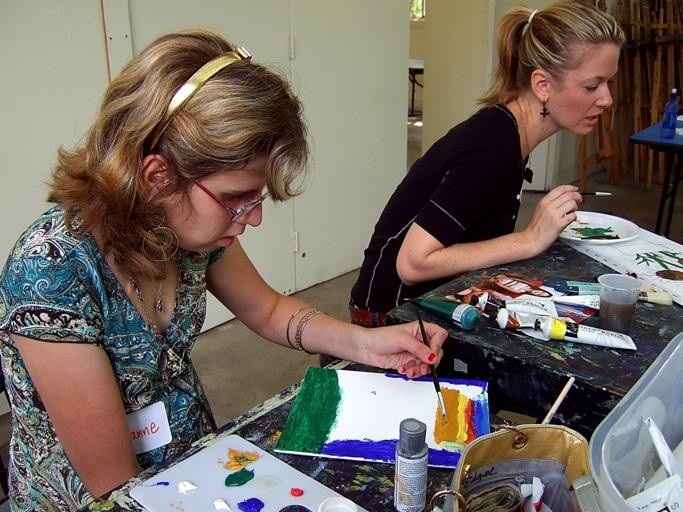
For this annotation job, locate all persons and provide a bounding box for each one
[349,1,625,323]
[0,30,448,512]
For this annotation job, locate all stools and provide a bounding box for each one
[409,68,424,117]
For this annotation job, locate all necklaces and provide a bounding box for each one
[145,275,167,314]
[514,96,530,164]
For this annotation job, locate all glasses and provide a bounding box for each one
[189,173,272,227]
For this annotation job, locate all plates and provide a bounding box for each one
[557,210,641,246]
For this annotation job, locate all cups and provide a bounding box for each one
[597,273,642,335]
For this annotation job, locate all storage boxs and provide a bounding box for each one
[587,331,683,512]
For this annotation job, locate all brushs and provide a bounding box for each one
[545,280,673,306]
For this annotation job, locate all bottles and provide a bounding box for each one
[660,88,680,140]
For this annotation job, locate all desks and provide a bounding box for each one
[77,221,683,512]
[629,113,683,238]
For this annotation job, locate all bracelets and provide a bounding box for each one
[285,305,313,349]
[295,308,330,355]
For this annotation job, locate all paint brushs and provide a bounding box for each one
[522,189,613,196]
[415,305,447,425]
